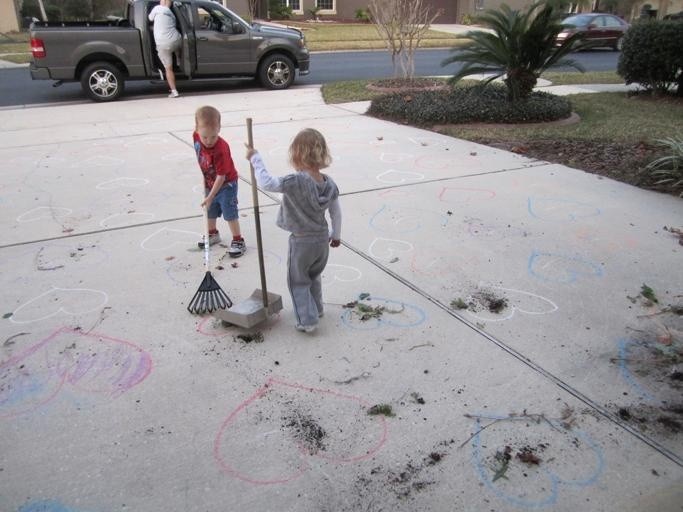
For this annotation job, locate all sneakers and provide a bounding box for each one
[198,230,220,247]
[168,90,178,97]
[229,238,246,256]
[295,324,320,332]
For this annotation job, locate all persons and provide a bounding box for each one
[191,106,247,258]
[147,0,183,98]
[244,128,342,333]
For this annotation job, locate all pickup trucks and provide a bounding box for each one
[29,0,310,102]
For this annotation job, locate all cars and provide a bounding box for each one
[554,12,631,53]
